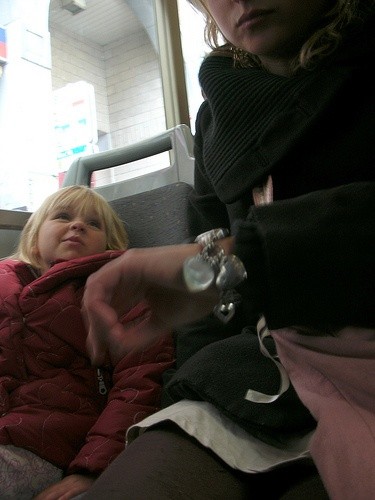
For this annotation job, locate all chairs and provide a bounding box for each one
[60,124,197,249]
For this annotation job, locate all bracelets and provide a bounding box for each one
[187,225,247,321]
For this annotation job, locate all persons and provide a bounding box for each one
[0,184,174,500]
[80,0,374,500]
